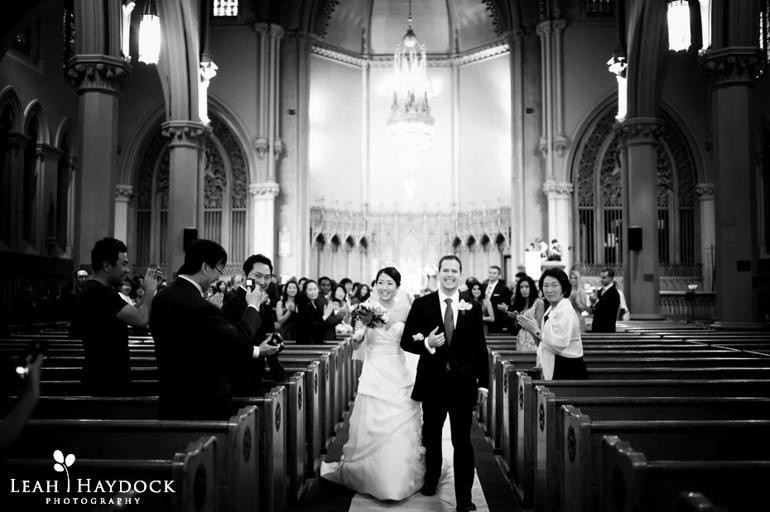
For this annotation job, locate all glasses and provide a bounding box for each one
[210,264,223,275]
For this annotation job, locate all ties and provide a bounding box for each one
[443,299,454,345]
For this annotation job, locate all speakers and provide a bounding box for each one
[182,228,197,252]
[627,226,643,252]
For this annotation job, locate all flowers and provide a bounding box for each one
[456,297,473,314]
[359,298,386,324]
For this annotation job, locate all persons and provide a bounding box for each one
[518,267,587,381]
[218,254,287,390]
[0,337,48,470]
[459,265,632,336]
[400,257,490,511]
[0,251,376,350]
[68,237,158,399]
[150,239,262,421]
[318,267,429,501]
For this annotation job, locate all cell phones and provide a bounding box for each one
[266,333,283,347]
[245,277,255,292]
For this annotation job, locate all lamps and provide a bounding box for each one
[665,0,692,53]
[138,2,162,68]
[391,1,430,123]
[602,50,629,82]
[197,0,220,89]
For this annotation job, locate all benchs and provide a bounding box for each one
[487,321,768,511]
[0,310,362,510]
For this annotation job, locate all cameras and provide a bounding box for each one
[147,263,162,278]
